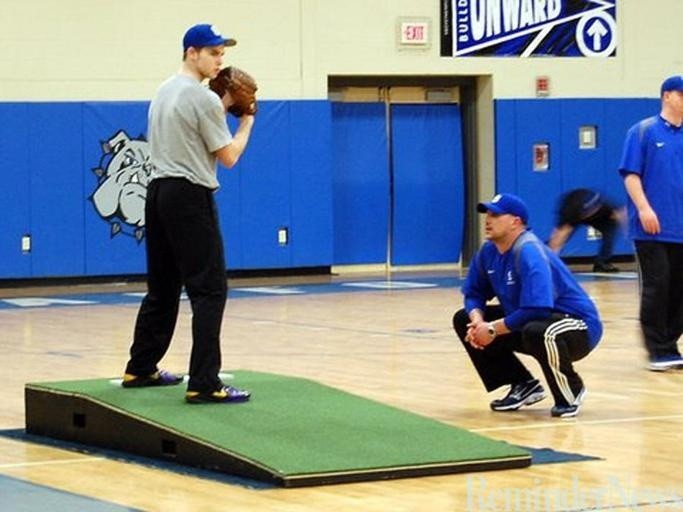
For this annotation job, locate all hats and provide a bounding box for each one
[477,193,529,225]
[184,25,235,49]
[661,77,683,94]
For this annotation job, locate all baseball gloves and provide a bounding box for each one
[209,66,257,117]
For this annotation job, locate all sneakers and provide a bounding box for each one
[186,383,249,401]
[122,369,183,387]
[552,385,586,418]
[491,379,546,410]
[648,353,683,370]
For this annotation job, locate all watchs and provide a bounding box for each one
[488,320,497,342]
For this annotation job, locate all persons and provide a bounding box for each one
[453,192,605,418]
[121,22,258,405]
[618,76,683,371]
[547,188,627,272]
